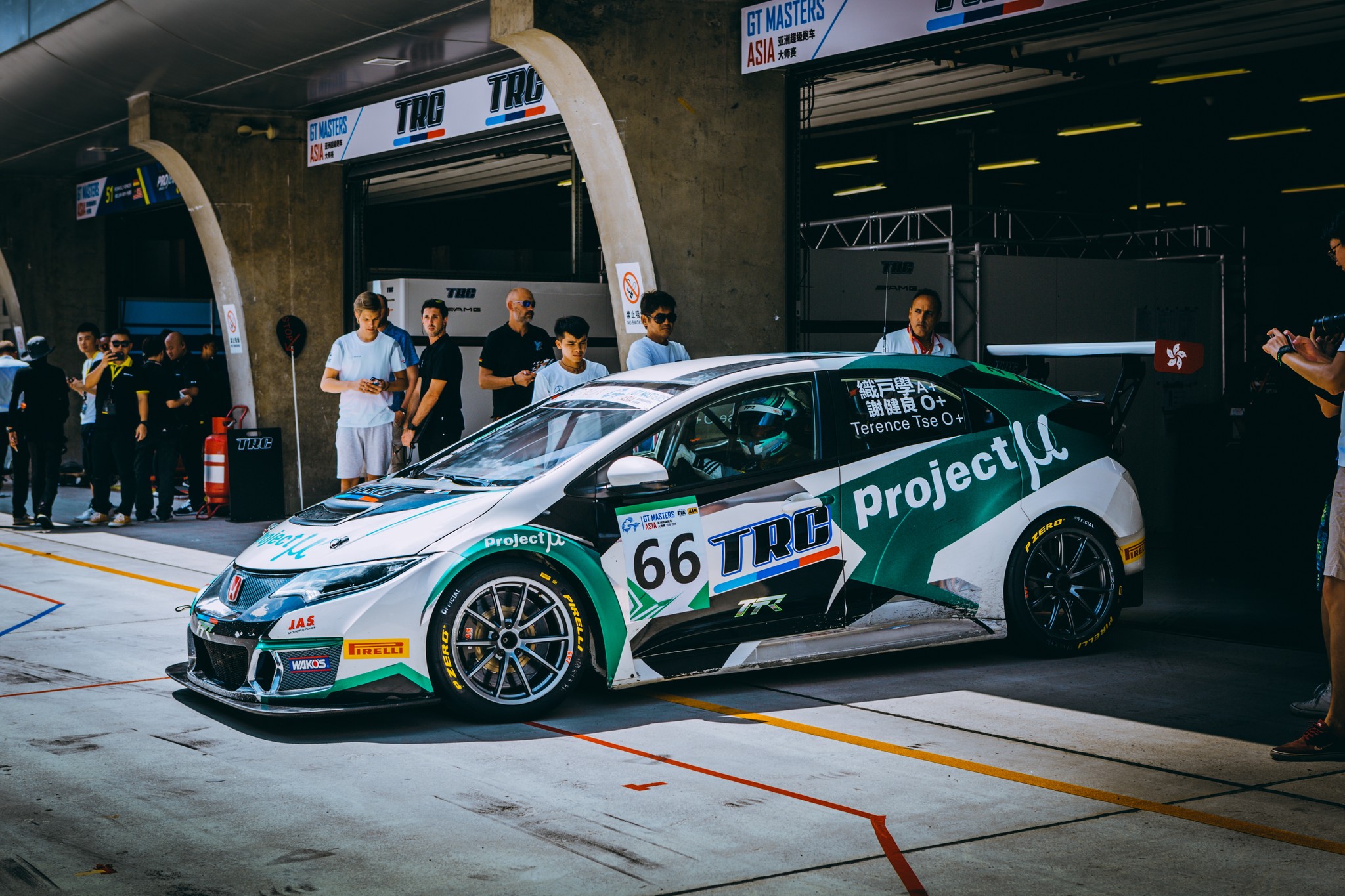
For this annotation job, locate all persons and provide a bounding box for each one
[672,392,804,480]
[98,330,232,522]
[873,287,958,358]
[626,290,691,451]
[82,327,150,527]
[0,340,36,524]
[8,336,70,534]
[1290,492,1332,715]
[1310,325,1344,418]
[400,298,465,462]
[65,327,115,522]
[376,294,420,476]
[1262,224,1345,760]
[530,315,612,471]
[479,286,557,464]
[319,291,409,493]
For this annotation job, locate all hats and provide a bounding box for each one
[21,336,55,362]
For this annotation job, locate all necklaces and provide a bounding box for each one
[561,359,584,375]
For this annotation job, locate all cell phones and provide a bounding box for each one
[107,352,124,361]
[1269,333,1296,350]
[12,436,18,452]
[365,377,382,391]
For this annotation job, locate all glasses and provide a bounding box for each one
[99,343,109,351]
[1327,242,1342,261]
[424,300,444,303]
[110,339,132,348]
[512,300,536,308]
[640,313,677,324]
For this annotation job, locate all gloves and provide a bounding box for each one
[673,444,722,480]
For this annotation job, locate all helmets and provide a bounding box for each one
[735,388,804,459]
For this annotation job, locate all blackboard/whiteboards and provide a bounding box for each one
[228,428,284,521]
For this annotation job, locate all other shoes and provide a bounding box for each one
[138,514,156,522]
[1270,718,1345,759]
[173,503,204,516]
[161,515,172,521]
[33,514,54,533]
[108,513,131,526]
[83,512,109,526]
[73,507,97,521]
[14,513,34,523]
[1289,678,1331,714]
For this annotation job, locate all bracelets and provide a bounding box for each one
[399,407,407,413]
[402,427,408,430]
[6,426,15,433]
[383,381,390,391]
[512,376,517,385]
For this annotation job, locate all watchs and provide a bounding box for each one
[1277,345,1292,367]
[140,420,149,427]
[407,423,416,431]
